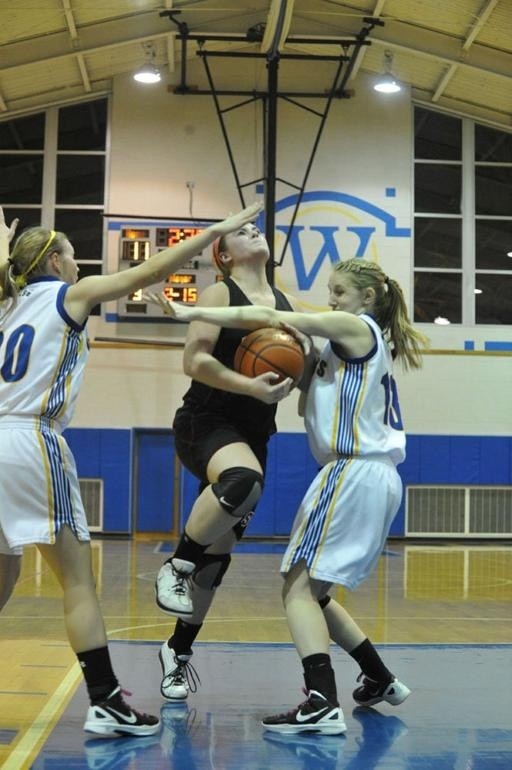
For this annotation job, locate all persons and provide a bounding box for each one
[140,256,431,735]
[1,204,266,741]
[156,222,320,703]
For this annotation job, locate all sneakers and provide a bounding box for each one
[262,730,346,765]
[159,702,200,759]
[353,673,412,706]
[157,635,201,702]
[352,706,408,745]
[152,557,195,619]
[83,735,159,769]
[262,690,346,735]
[83,704,162,736]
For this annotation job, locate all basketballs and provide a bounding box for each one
[234,329,305,392]
